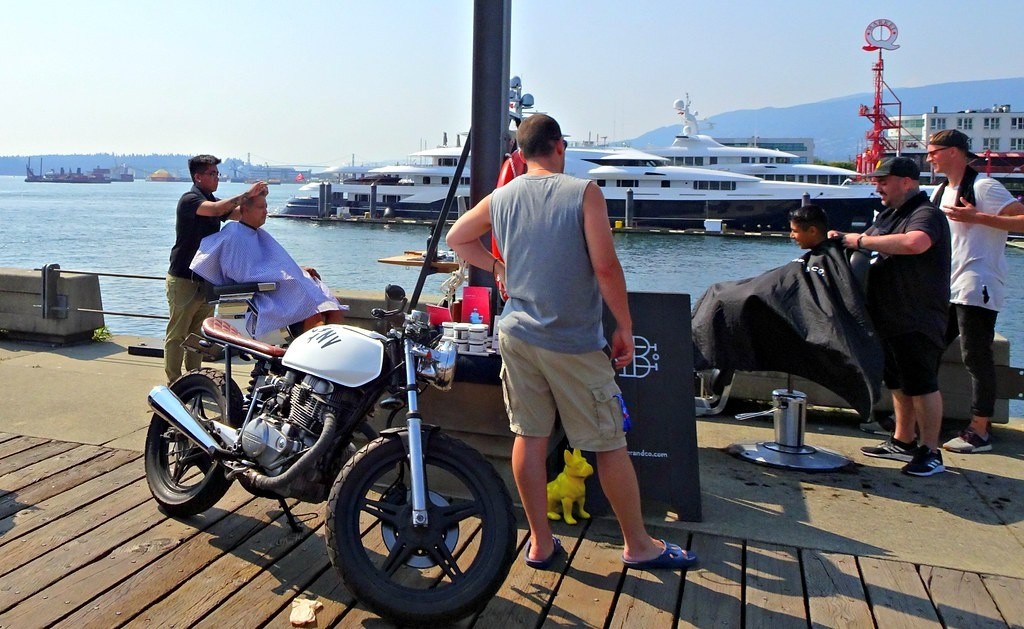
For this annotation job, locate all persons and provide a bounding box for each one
[826,156,952,477]
[446,113,699,569]
[220,187,344,334]
[906,142,918,147]
[882,142,887,148]
[859,104,869,114]
[163,155,268,386]
[787,204,831,253]
[924,129,1024,455]
[866,147,872,155]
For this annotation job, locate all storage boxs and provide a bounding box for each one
[461,285,493,332]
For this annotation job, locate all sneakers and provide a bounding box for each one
[860,437,917,462]
[900,445,945,475]
[944,430,993,454]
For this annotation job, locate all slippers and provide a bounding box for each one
[621,539,699,568]
[526,534,562,568]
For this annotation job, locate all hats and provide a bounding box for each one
[862,157,920,180]
[930,129,980,158]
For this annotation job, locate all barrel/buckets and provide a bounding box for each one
[426,305,451,326]
[615,221,622,228]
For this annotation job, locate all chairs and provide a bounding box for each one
[694,240,878,477]
[194,234,329,351]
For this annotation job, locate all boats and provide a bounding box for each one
[24,166,111,183]
[266,75,940,235]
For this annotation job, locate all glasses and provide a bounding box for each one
[928,145,951,158]
[200,173,221,178]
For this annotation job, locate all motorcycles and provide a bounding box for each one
[143,284,519,629]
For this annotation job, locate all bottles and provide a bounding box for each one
[427,226,437,262]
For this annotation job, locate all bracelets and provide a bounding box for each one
[857,236,864,249]
[493,258,499,273]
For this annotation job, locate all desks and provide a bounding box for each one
[358,351,566,508]
[378,250,459,274]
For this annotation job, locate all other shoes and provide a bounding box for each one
[858,414,897,436]
